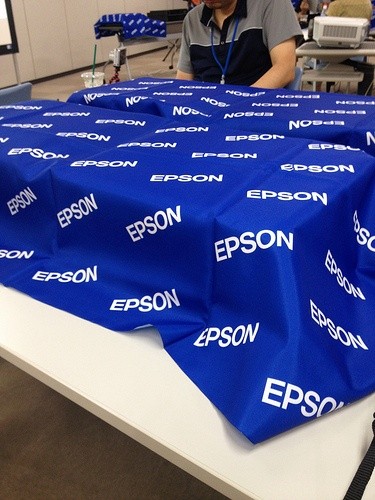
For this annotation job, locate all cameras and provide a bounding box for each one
[109,47,126,67]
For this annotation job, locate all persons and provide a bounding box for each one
[176,0,304,90]
[291,0,375,39]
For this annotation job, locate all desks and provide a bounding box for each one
[99,21,183,82]
[295,42,375,96]
[0,281,375,500]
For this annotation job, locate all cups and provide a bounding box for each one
[80,72,105,88]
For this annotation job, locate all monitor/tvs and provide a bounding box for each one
[0,0,19,56]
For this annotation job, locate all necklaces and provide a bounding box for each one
[210,18,239,85]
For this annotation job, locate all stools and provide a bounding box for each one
[301,71,364,94]
